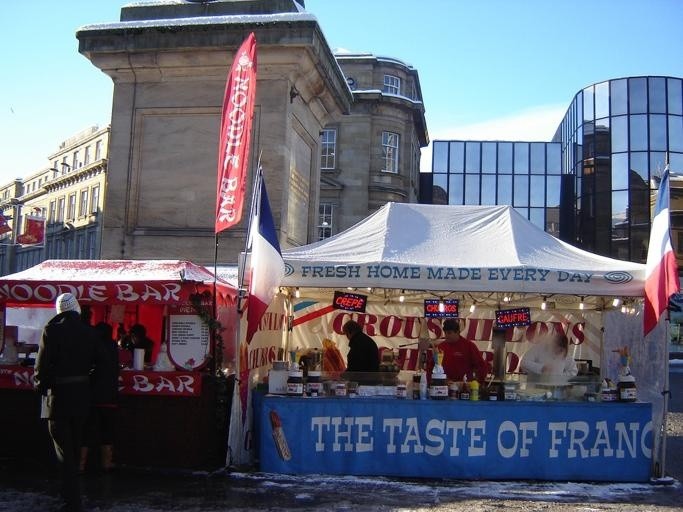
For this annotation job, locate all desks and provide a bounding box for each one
[257,392,653,482]
[0,375,217,468]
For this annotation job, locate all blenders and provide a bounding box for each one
[574,358,599,378]
[268,360,294,394]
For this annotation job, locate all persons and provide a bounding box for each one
[121,324,154,363]
[34,292,119,512]
[436,318,488,386]
[521,335,579,381]
[340,321,381,385]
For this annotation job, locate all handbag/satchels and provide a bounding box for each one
[40,389,54,419]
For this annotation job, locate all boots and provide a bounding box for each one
[79,447,89,471]
[99,445,117,472]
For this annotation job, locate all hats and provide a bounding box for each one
[56,292,81,314]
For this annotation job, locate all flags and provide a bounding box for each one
[643,164,681,337]
[246,165,285,345]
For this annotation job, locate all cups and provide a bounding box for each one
[134,348,145,370]
